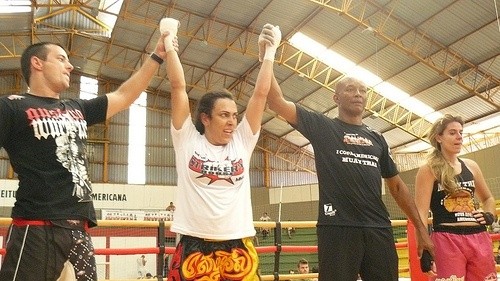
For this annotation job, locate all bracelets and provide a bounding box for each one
[150,52,164,64]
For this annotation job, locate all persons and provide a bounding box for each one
[258,23,436,281]
[259,212,272,240]
[289,258,311,281]
[286,221,297,240]
[159,18,282,281]
[414,114,499,281]
[136,254,165,281]
[166,202,176,211]
[0,31,180,281]
